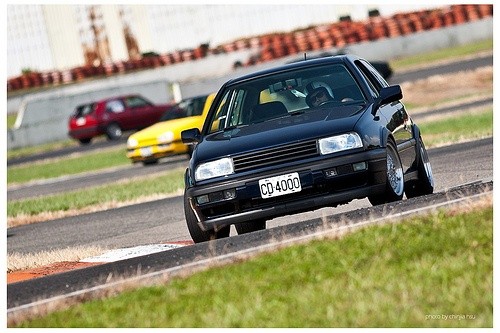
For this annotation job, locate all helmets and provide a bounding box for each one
[303,83,335,108]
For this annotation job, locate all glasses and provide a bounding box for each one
[309,91,324,103]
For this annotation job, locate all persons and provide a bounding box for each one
[302,81,354,108]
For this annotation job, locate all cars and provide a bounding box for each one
[283,54,393,90]
[69,92,178,143]
[182,53,434,242]
[126,91,274,165]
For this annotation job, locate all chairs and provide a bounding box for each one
[331,84,364,102]
[248,100,288,124]
